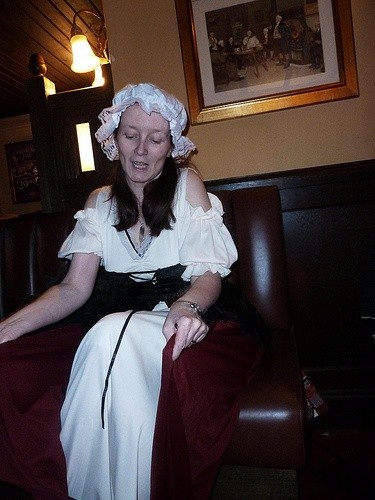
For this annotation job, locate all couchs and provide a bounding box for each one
[0,185,306,500]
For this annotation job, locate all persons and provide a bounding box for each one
[0,83,268,500]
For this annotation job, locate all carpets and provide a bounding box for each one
[298,428,375,500]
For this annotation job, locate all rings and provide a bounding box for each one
[190,340,196,344]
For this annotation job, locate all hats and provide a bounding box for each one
[96,82,197,167]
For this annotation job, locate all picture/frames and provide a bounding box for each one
[174,0,360,126]
[5,140,40,205]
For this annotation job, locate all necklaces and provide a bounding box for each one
[137,217,148,244]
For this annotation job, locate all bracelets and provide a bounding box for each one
[170,299,203,317]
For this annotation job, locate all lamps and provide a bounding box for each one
[70,7,110,74]
